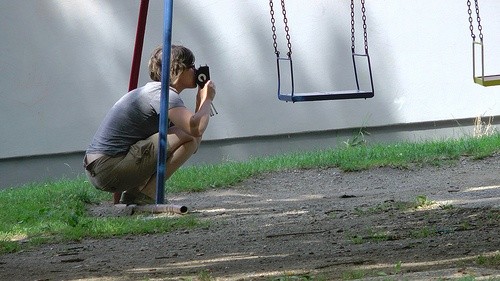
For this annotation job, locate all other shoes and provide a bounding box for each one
[120,191,155,204]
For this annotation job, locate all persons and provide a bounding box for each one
[84,46,216,206]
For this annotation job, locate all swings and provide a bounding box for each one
[467,0,500,86]
[269,0,375,102]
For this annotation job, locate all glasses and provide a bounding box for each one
[185,63,195,69]
